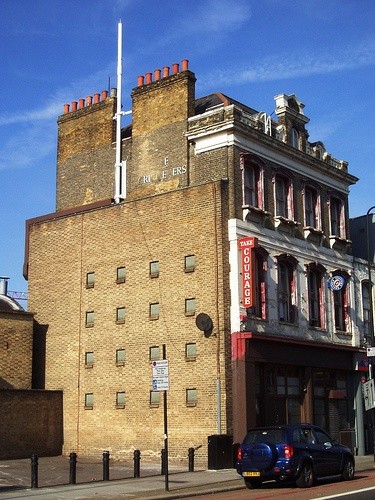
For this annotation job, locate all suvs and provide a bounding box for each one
[235,422,354,487]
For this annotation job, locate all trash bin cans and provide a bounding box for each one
[208,434,234,470]
